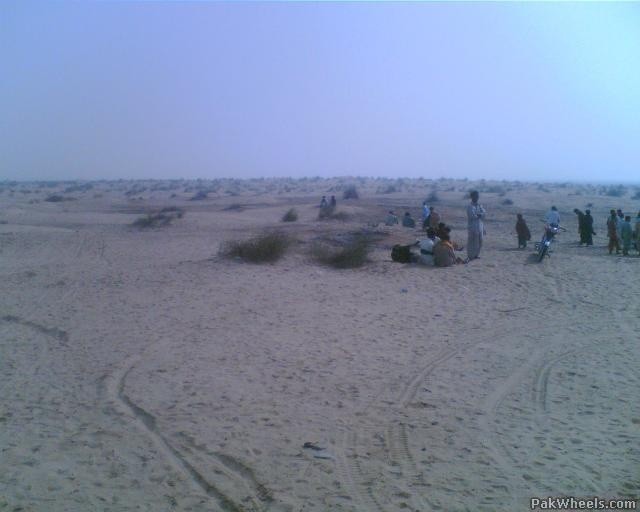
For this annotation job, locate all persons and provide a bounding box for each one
[463,188,489,263]
[514,213,532,249]
[544,205,561,227]
[320,195,328,209]
[329,195,336,208]
[383,200,470,268]
[572,206,640,257]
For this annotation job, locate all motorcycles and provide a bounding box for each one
[535,218,567,263]
